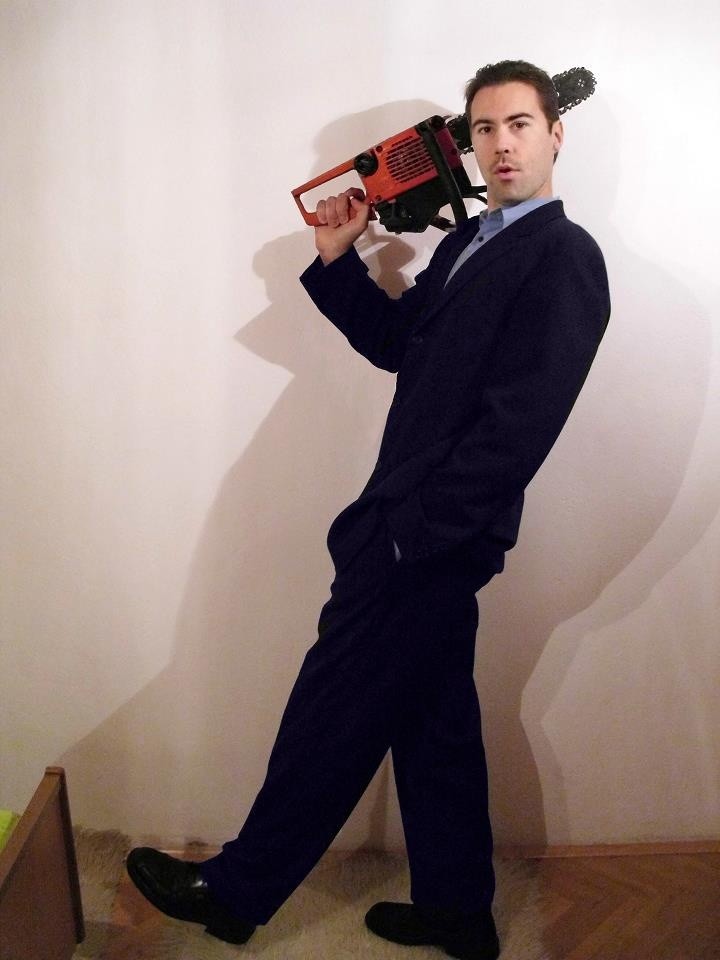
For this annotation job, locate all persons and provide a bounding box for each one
[124,61,610,960]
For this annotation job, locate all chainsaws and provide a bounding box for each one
[291,67,598,233]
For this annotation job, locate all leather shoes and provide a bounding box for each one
[126,847,255,944]
[365,901,500,960]
